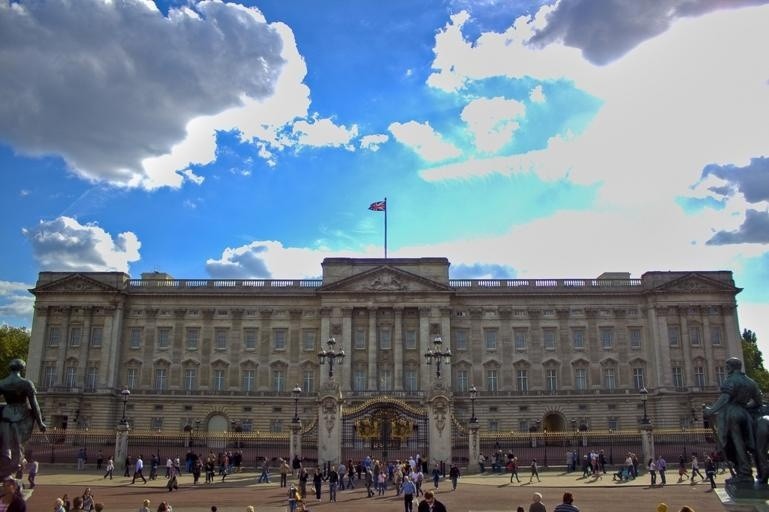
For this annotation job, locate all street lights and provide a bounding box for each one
[230,418,236,438]
[467,385,478,423]
[535,419,541,434]
[193,417,200,437]
[571,416,576,431]
[317,334,345,380]
[637,384,650,423]
[290,383,302,425]
[117,385,131,427]
[423,334,453,380]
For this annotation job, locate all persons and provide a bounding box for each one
[0,359,47,483]
[28,457,39,489]
[702,356,765,485]
[56,442,718,511]
[4,480,27,512]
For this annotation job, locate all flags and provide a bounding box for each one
[368,201,386,211]
